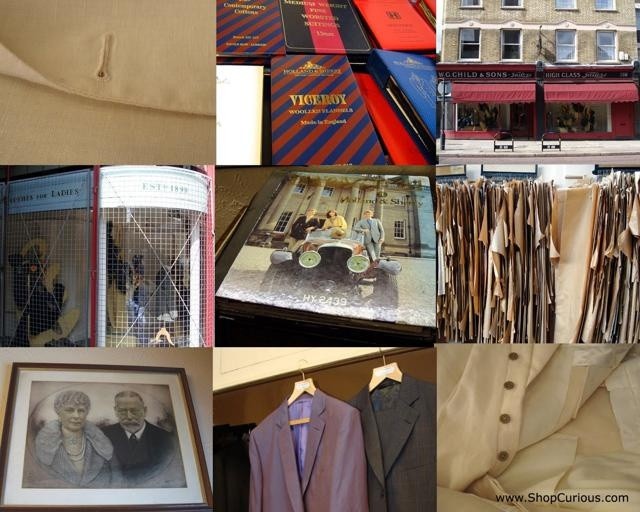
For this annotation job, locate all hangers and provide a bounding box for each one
[285,358,316,426]
[364,348,406,393]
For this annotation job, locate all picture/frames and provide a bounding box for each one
[0,361,214,512]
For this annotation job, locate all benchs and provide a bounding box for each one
[542,131,561,152]
[493,132,515,152]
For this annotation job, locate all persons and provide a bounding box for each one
[352,211,385,261]
[318,209,349,230]
[34,390,113,488]
[103,391,173,480]
[287,207,318,253]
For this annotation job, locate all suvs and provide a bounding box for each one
[260,231,403,310]
